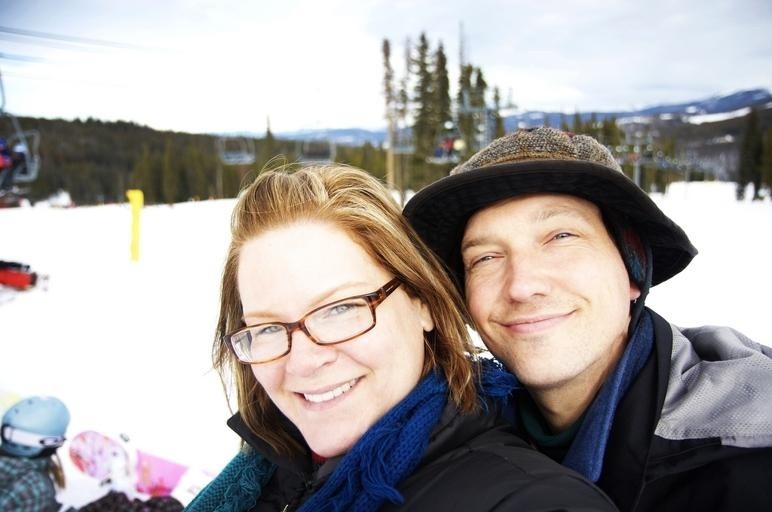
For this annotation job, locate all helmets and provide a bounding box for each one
[1,397,69,457]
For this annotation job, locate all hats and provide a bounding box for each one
[401,127,699,288]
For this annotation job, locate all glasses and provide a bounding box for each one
[223,276,403,365]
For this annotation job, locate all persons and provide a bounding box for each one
[181,159,622,511]
[734,111,769,202]
[1,394,83,512]
[402,124,772,512]
